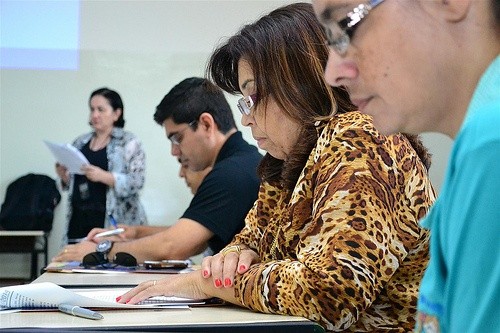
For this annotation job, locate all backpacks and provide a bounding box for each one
[0,174,62,233]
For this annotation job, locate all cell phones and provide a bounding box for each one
[143,262,187,269]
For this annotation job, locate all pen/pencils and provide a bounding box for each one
[58,304,104,319]
[81,228,124,241]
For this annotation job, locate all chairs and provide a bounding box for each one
[0,173,62,285]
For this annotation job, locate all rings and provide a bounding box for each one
[154,280,156,285]
[65,249,67,254]
[223,246,241,258]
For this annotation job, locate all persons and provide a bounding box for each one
[109,157,213,257]
[55,87,149,250]
[312,0,500,333]
[52,76,263,264]
[116,3,439,333]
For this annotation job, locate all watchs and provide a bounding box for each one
[96,240,115,259]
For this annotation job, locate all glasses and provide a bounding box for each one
[237,88,272,116]
[169,119,197,146]
[325,0,382,55]
[79,251,138,271]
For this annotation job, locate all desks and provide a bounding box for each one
[0,262,326,333]
